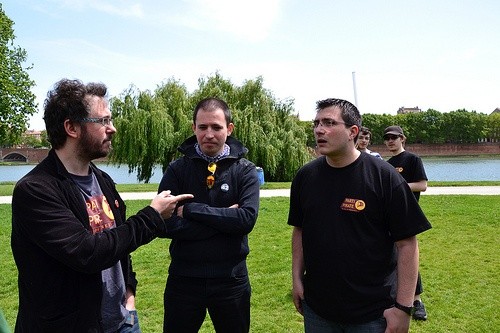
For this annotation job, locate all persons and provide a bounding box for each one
[158,99,259,333]
[286,98,433,333]
[354,124,428,321]
[10,79,195,333]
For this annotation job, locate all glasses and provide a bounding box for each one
[206,161,217,190]
[311,118,352,127]
[81,116,113,125]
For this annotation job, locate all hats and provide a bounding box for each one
[384,125,404,137]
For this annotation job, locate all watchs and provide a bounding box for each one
[394,302,415,316]
